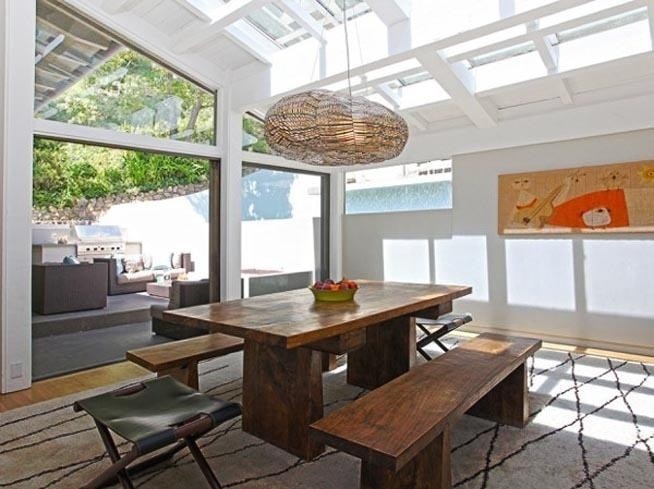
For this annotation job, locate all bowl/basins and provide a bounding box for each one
[307,285,358,301]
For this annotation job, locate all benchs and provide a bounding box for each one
[127,333,339,391]
[309,332,543,486]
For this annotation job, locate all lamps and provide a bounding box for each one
[263,0,409,167]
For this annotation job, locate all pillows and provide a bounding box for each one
[104,253,182,275]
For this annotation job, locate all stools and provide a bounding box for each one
[416,316,472,361]
[74,374,242,488]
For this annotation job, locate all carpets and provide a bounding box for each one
[0,330,654,489]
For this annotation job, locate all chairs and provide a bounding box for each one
[33,262,108,315]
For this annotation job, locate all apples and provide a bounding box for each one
[313,279,359,290]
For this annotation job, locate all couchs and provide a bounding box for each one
[95,253,191,296]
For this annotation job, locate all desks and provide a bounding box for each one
[164,280,471,464]
